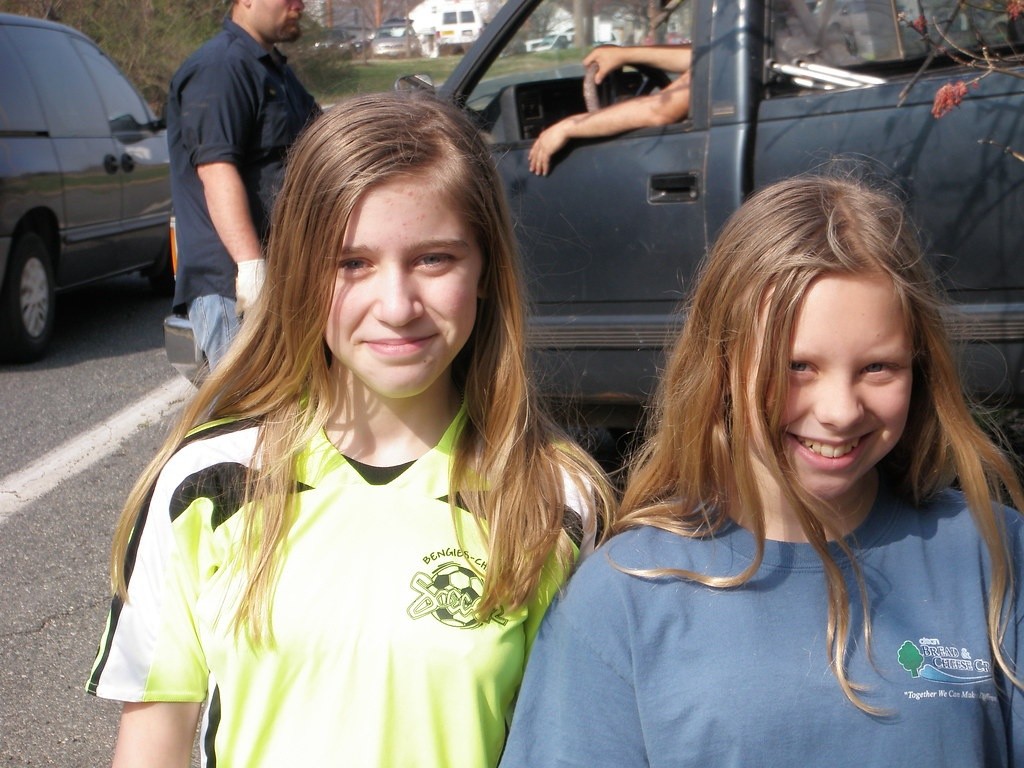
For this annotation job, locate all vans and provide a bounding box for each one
[1,12,176,365]
[435,4,484,44]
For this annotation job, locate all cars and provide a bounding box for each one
[313,29,356,60]
[368,19,421,59]
[533,32,575,52]
[163,1,1024,513]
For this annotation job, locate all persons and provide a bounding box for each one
[494,177,1023,768]
[85,93,614,768]
[528,42,692,177]
[165,0,326,374]
